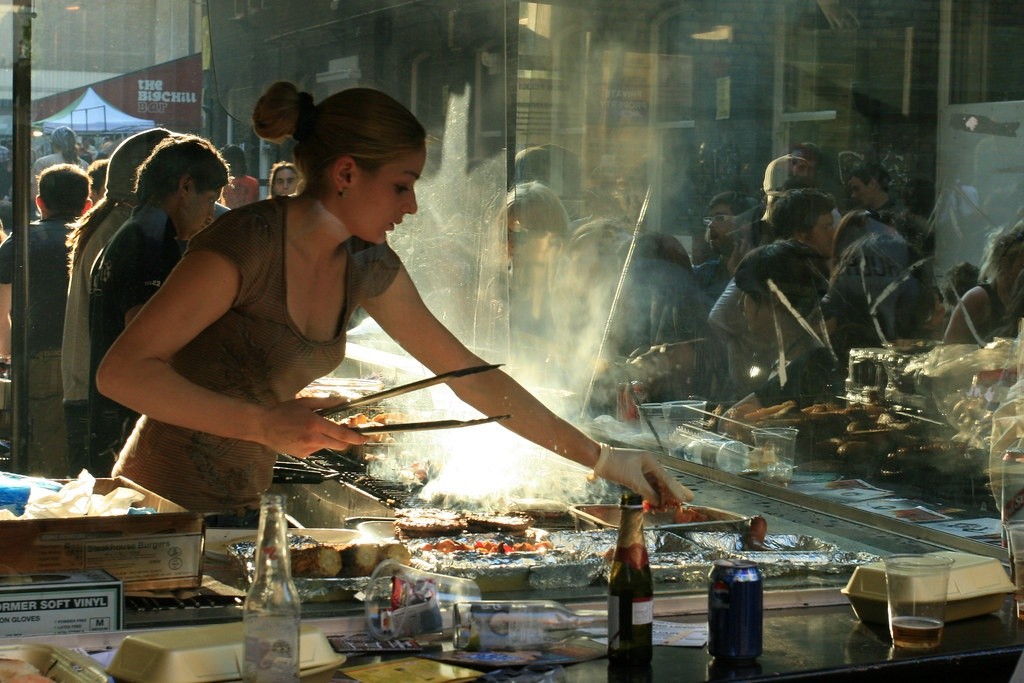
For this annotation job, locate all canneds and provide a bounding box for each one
[708,557,762,659]
[615,380,643,424]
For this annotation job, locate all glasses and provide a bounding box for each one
[702,215,739,227]
[502,225,552,246]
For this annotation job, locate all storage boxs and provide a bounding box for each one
[0,476,207,589]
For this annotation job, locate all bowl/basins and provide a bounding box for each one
[840,547,1020,630]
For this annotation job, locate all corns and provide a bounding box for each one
[291,542,411,577]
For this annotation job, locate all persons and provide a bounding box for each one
[404,145,1023,401]
[0,163,90,475]
[222,145,260,210]
[97,85,694,531]
[0,126,116,246]
[270,163,300,196]
[85,135,229,477]
[62,129,175,477]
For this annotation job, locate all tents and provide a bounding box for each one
[30,86,154,139]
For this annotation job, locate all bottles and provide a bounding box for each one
[241,495,301,682]
[607,493,653,670]
[451,600,608,651]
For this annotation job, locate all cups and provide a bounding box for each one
[751,427,799,487]
[363,560,481,640]
[636,403,666,453]
[662,401,707,459]
[883,552,954,649]
[1003,520,1024,621]
[692,440,751,473]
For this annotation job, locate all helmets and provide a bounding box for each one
[103,126,175,202]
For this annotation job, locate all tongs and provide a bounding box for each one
[314,362,511,431]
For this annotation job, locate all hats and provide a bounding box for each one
[50,126,76,149]
[0,146,11,163]
[516,143,584,197]
[759,153,820,221]
